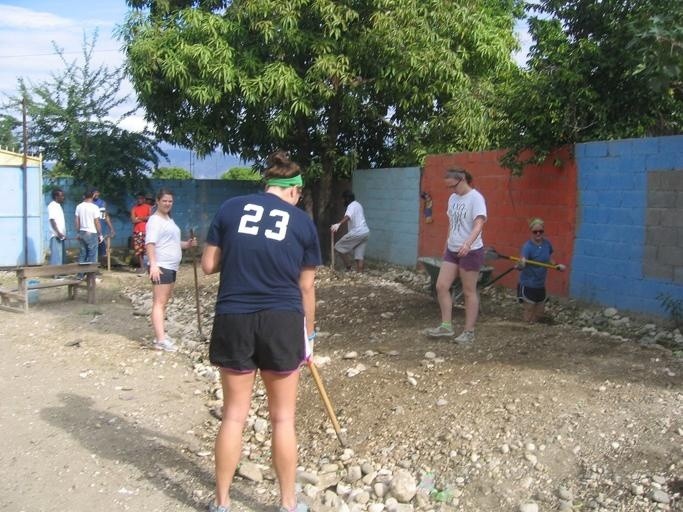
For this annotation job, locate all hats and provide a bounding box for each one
[144,194,157,202]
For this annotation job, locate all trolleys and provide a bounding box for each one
[417,256,516,309]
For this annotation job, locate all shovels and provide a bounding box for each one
[484,246,560,270]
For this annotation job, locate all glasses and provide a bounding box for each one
[445,177,465,190]
[530,228,545,235]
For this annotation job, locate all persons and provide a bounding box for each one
[93,188,115,268]
[426,167,488,345]
[201,153,322,512]
[517,218,566,325]
[75,187,104,267]
[331,191,369,274]
[47,188,66,273]
[132,193,153,272]
[145,188,198,353]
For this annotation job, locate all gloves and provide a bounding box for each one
[98,234,104,245]
[302,330,320,362]
[554,262,567,273]
[329,222,342,234]
[514,255,527,271]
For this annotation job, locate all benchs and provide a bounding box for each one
[0,262,100,312]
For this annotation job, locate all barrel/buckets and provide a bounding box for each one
[26,280,41,305]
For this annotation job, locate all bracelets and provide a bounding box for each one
[309,332,318,340]
[136,217,138,219]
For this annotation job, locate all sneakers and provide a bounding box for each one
[426,323,455,337]
[207,498,230,512]
[452,331,477,346]
[278,502,308,512]
[153,334,177,345]
[155,341,180,353]
